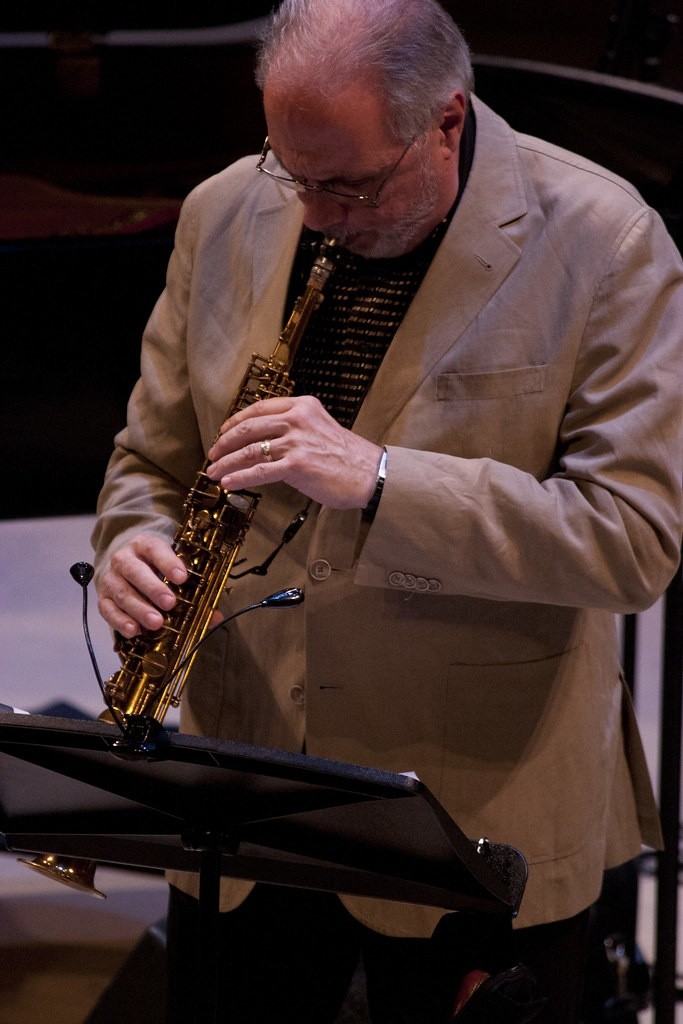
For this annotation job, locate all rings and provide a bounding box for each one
[260,438,272,460]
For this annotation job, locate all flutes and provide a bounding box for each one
[14,233,350,899]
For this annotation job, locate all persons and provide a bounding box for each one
[89,0,683,1024]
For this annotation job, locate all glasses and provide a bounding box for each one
[256,128,417,205]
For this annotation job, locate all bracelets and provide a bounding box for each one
[362,448,392,518]
